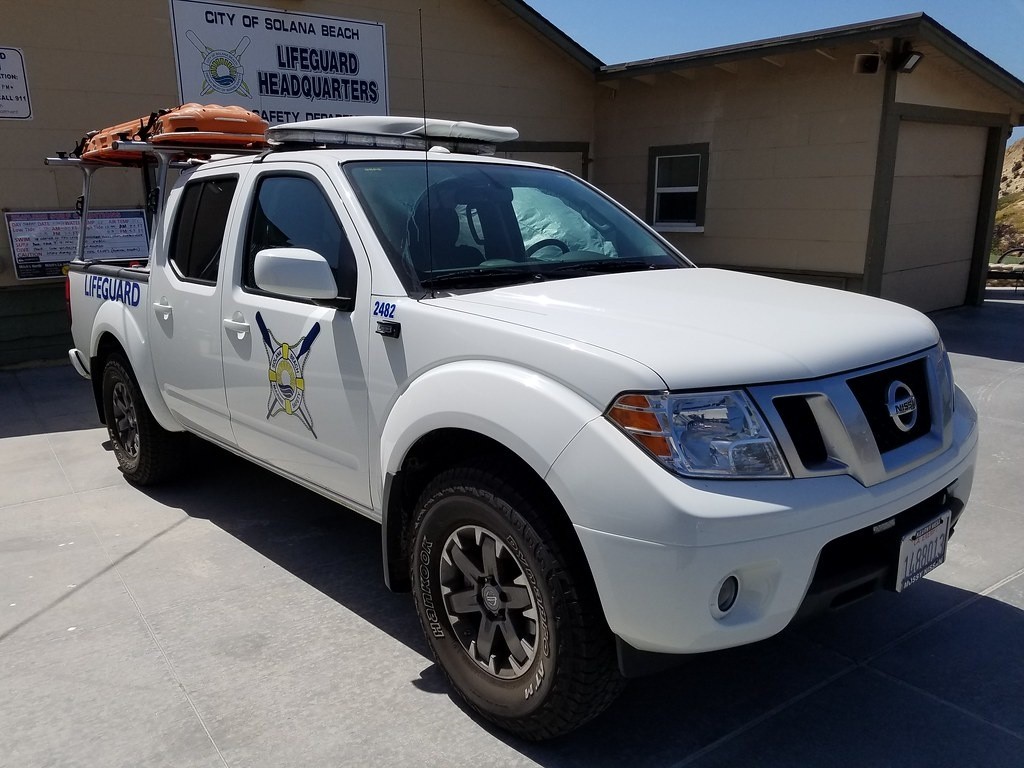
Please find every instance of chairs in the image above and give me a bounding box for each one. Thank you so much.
[406,206,486,282]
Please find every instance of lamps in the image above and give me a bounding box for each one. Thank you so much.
[900,40,926,74]
[852,46,890,74]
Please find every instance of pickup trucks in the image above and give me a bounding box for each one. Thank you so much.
[45,102,981,747]
